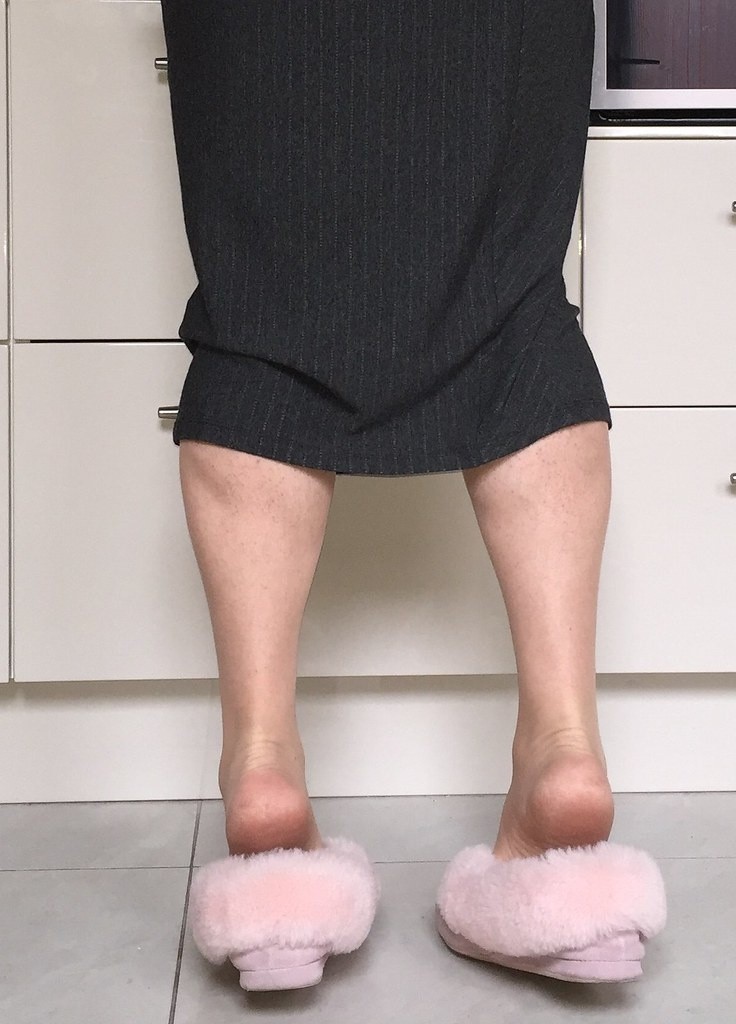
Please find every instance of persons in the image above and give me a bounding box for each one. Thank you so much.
[159,0,668,860]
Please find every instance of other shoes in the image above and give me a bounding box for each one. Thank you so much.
[434,841,666,984]
[193,837,377,990]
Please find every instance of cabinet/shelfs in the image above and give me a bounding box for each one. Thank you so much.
[0,0,736,806]
